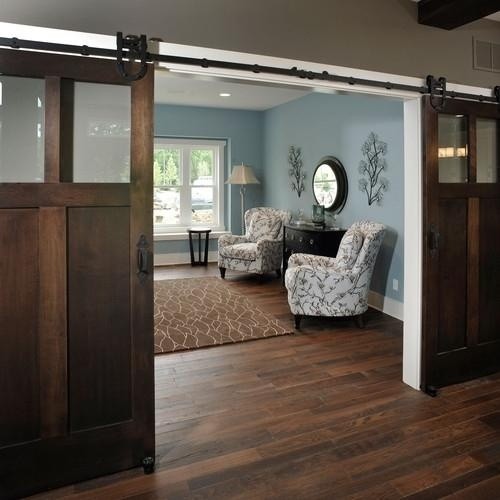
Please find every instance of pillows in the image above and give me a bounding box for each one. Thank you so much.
[246,211,282,243]
[333,224,364,270]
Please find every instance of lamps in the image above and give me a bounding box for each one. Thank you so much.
[224,162,261,235]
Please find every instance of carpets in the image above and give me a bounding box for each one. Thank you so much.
[154,276,295,355]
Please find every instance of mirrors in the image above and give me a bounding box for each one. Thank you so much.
[312,156,348,217]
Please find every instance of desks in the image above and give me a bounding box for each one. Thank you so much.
[187,228,211,267]
[283,222,347,286]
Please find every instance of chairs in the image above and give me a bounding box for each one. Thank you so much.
[285,220,387,332]
[218,207,293,282]
